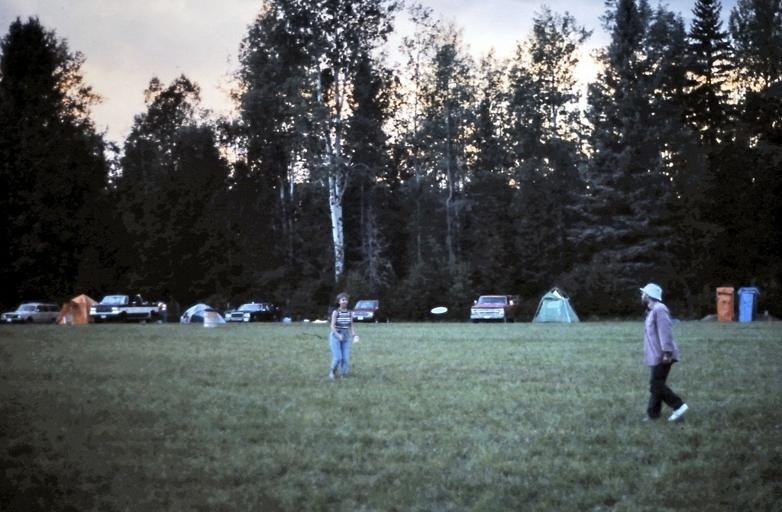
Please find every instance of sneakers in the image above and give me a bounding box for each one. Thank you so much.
[668,403,688,421]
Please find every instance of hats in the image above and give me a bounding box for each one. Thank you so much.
[640,283,662,302]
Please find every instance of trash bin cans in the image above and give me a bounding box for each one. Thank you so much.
[717,287,734,322]
[737,287,760,322]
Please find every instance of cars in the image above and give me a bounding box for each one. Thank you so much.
[352,300,384,321]
[225,302,275,322]
[470,294,517,321]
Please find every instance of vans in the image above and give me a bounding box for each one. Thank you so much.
[3,302,59,323]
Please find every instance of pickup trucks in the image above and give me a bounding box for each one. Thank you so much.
[89,294,161,324]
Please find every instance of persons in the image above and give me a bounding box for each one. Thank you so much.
[638,282,690,424]
[329,291,359,380]
[132,293,149,324]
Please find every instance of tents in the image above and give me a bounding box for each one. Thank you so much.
[531,286,580,323]
[55,293,99,324]
[179,303,227,325]
